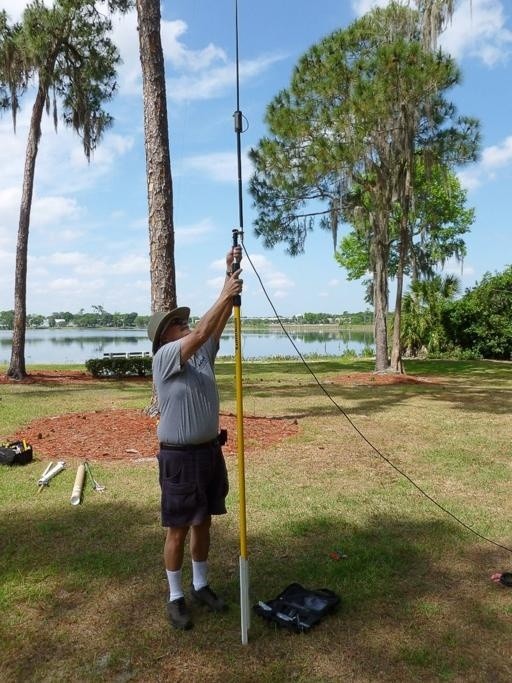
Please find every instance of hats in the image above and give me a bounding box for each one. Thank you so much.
[147,305,190,356]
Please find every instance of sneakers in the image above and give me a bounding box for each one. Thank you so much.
[166,592,194,630]
[190,581,229,612]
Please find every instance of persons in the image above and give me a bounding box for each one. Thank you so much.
[147,243,244,631]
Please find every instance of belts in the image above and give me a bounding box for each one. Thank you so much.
[160,436,223,451]
